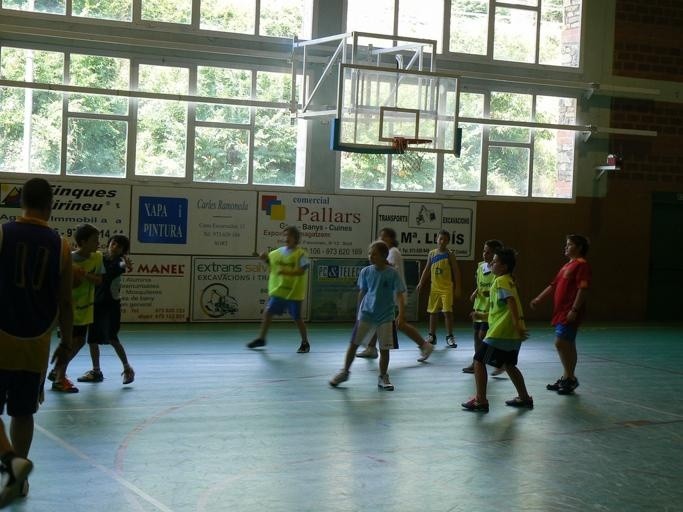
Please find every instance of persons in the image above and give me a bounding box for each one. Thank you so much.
[415,229,462,348]
[462,238,505,376]
[326,238,407,392]
[529,232,592,395]
[78,233,134,385]
[0,176,77,511]
[461,245,534,412]
[245,224,311,354]
[45,221,136,394]
[355,227,436,362]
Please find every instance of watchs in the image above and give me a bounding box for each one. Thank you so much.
[571,306,579,313]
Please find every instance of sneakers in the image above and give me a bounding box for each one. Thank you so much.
[331,369,350,385]
[417,344,432,361]
[461,396,489,411]
[121,368,134,384]
[379,374,394,389]
[0,452,33,507]
[463,362,476,372]
[77,371,104,382]
[505,396,533,408]
[357,346,377,357]
[547,377,579,393]
[48,369,79,393]
[297,343,310,354]
[246,338,265,348]
[425,333,457,348]
[491,368,506,375]
